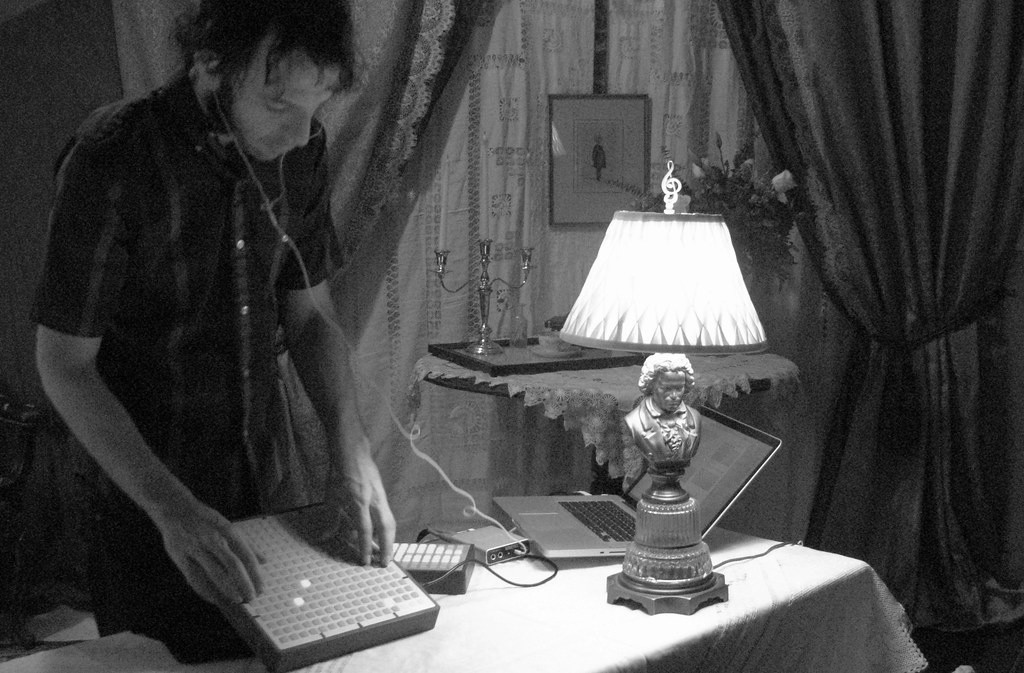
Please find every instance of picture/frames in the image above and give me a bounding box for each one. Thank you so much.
[547,92,651,227]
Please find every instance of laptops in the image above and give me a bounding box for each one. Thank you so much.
[492,406,782,558]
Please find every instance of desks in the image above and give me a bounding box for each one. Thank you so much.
[0,526,872,673]
[419,353,798,495]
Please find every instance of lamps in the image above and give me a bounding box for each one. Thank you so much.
[553,156,770,613]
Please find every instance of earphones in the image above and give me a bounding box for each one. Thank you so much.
[207,60,217,73]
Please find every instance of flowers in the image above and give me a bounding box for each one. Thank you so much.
[648,129,808,294]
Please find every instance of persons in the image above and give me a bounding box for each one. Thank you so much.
[625,351,700,470]
[34,0,395,658]
